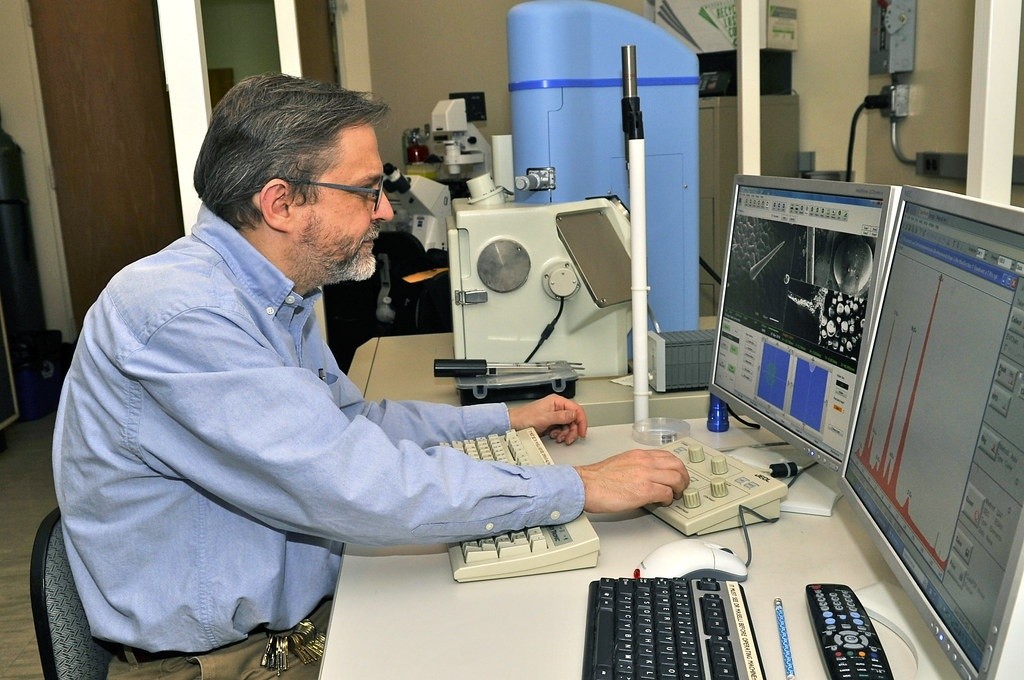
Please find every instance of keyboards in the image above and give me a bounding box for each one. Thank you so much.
[581,578,767,680]
[439,426,602,582]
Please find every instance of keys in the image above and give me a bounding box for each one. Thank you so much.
[260,620,327,677]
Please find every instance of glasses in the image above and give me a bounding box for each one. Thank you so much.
[250,177,384,212]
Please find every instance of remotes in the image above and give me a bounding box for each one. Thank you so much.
[806,584,894,680]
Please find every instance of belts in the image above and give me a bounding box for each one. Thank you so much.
[112,644,190,663]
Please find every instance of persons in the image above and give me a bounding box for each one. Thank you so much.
[53,73,691,680]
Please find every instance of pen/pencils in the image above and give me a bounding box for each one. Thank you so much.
[773,597,795,679]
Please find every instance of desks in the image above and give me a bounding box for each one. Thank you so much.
[320,415,1024,680]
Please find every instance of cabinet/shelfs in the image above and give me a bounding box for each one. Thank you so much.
[699,95,798,315]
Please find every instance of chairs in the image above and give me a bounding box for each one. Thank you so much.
[30,506,112,680]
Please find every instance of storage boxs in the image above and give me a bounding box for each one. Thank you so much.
[694,48,794,101]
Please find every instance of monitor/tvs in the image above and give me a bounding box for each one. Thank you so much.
[835,184,1024,680]
[710,174,904,517]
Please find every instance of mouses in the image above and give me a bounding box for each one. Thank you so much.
[633,538,748,582]
